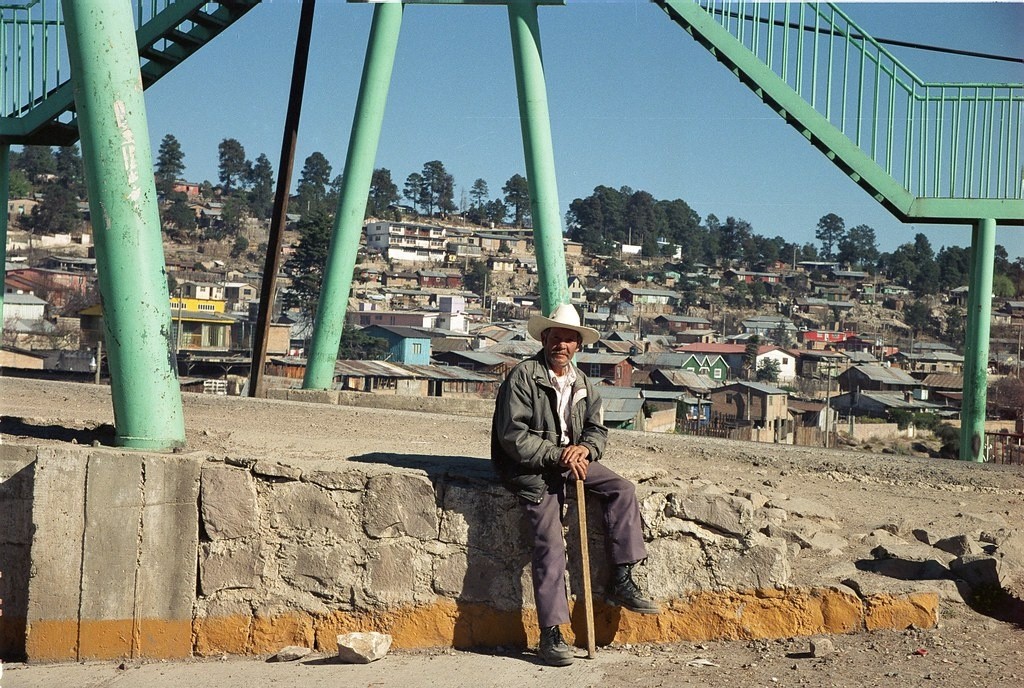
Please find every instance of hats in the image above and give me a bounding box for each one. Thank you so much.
[527,303,600,345]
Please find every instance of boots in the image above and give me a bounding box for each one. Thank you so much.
[539,626,574,666]
[606,565,660,613]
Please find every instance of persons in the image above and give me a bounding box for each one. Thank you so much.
[491,304,661,665]
